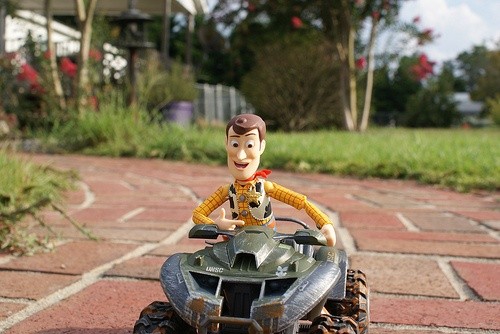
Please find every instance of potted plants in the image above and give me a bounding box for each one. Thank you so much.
[148,54,198,127]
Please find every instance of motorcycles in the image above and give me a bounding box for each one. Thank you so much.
[133,216,371,334]
